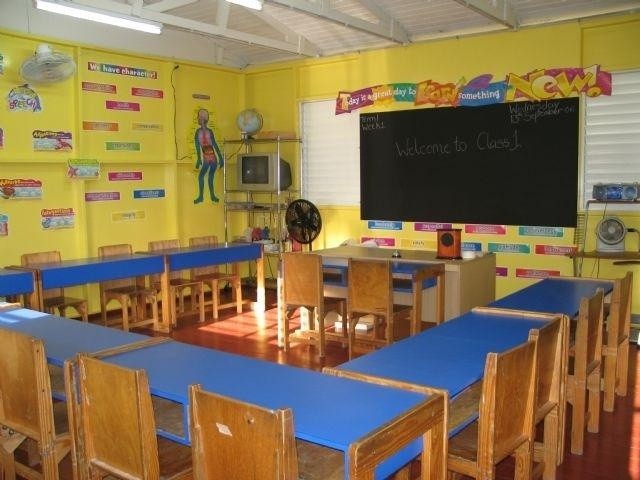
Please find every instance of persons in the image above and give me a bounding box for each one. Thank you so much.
[190,106,226,207]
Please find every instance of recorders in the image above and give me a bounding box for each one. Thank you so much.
[592,182,640,203]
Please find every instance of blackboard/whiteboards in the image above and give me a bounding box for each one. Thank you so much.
[359,96,579,228]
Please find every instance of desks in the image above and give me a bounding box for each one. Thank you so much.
[135,240,269,318]
[10,250,177,333]
[318,307,562,397]
[62,334,445,479]
[274,253,447,354]
[1,300,159,373]
[471,274,618,314]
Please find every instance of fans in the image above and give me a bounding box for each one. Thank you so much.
[21,42,74,86]
[285,198,322,252]
[594,216,626,253]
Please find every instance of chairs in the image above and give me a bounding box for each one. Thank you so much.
[189,236,245,321]
[0,328,67,478]
[447,315,568,480]
[345,257,414,358]
[96,242,161,336]
[181,380,300,480]
[20,250,89,322]
[568,287,608,459]
[602,269,632,415]
[279,252,346,356]
[70,353,189,479]
[145,238,209,327]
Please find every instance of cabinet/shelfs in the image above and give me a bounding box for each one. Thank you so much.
[223,135,303,281]
[571,200,640,324]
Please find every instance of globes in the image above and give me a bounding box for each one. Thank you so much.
[236,107,264,140]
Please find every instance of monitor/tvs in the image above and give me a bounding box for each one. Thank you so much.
[237,152,292,191]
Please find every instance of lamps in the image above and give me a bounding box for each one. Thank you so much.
[32,0,165,35]
